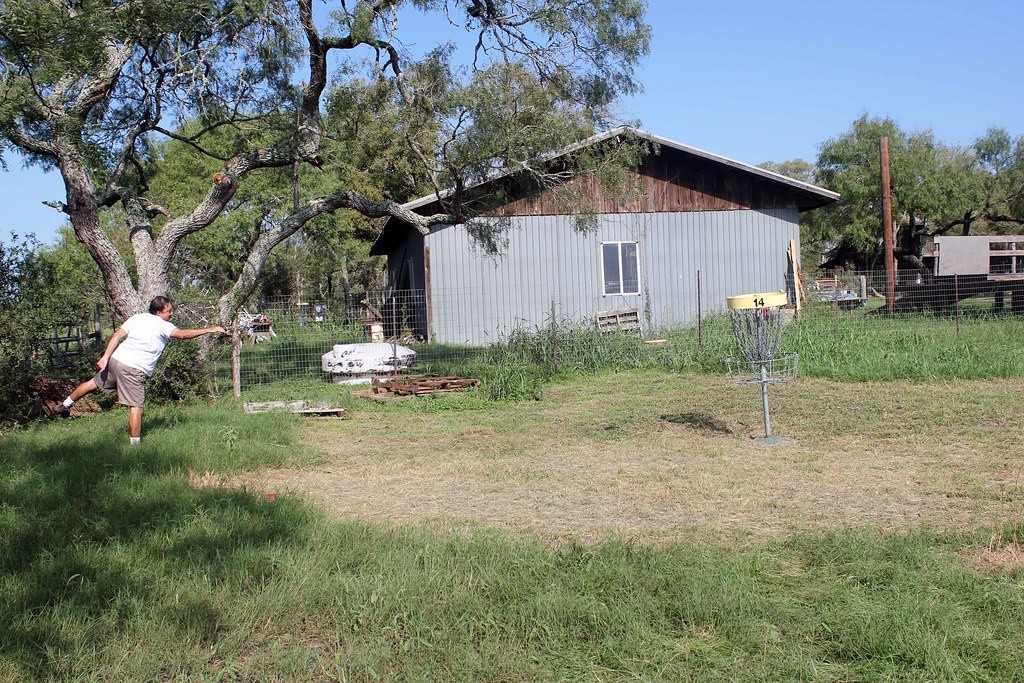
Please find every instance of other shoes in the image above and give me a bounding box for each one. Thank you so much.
[53,405,70,418]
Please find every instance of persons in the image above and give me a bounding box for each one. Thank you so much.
[53,295,227,445]
